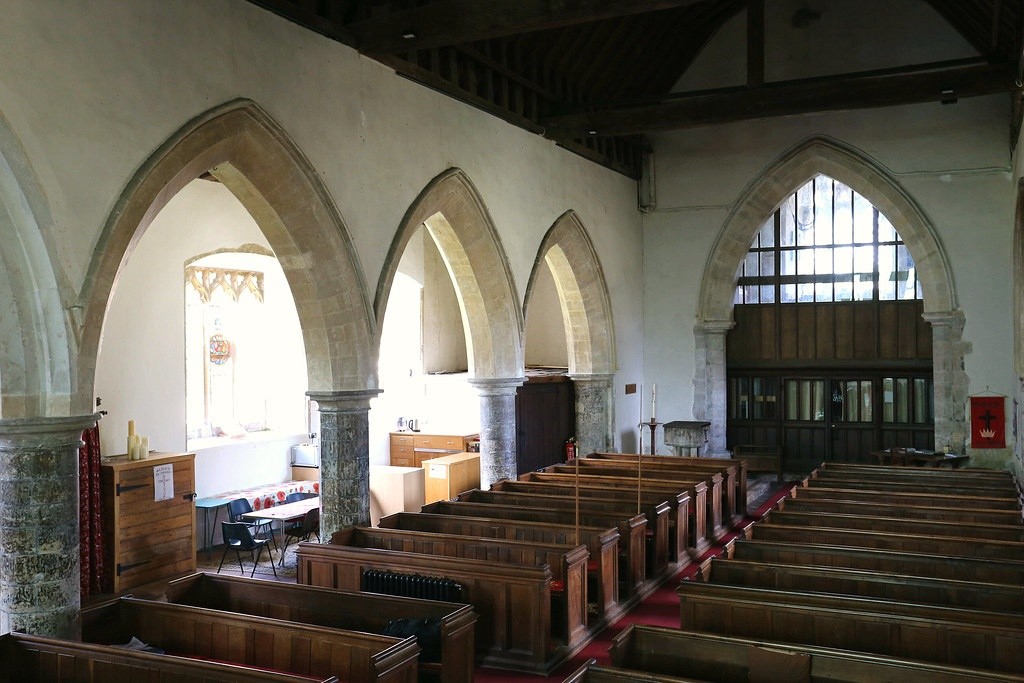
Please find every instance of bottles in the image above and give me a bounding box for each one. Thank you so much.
[127,435,134,460]
[398,417,407,432]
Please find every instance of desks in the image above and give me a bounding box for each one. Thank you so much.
[241,497,322,567]
[872,447,968,470]
[195,499,231,560]
[208,481,320,548]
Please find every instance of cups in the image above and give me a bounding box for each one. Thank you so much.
[409,418,421,432]
[132,444,140,460]
[140,445,148,459]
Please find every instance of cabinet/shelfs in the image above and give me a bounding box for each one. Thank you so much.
[100,453,197,595]
[292,466,320,480]
[369,430,480,528]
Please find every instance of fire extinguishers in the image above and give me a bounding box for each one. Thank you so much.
[565,436,575,463]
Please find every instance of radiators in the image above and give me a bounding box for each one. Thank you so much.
[361,571,465,604]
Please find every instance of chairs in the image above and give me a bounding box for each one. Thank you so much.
[217,492,320,578]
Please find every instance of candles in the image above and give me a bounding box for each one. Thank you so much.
[652,383,655,417]
[126,421,150,461]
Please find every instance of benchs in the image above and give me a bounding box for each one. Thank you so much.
[0,452,1024,683]
